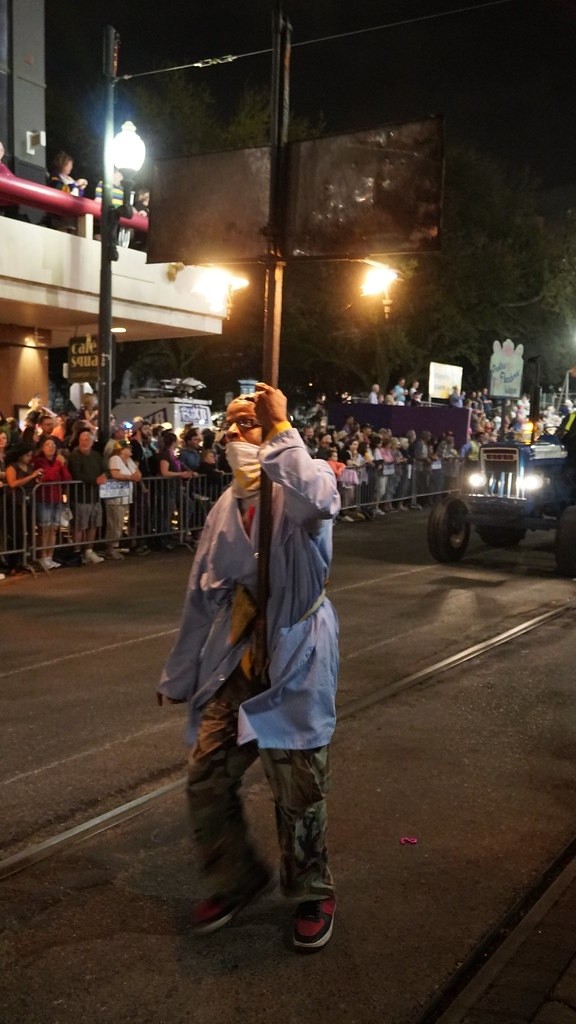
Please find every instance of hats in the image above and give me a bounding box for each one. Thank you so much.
[360,423,374,428]
[113,439,134,449]
[132,416,144,424]
[446,430,456,437]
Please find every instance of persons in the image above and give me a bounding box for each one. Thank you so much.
[449,384,576,464]
[95,170,150,248]
[301,415,459,527]
[48,150,88,233]
[0,141,16,218]
[154,382,342,946]
[368,378,424,407]
[0,394,234,581]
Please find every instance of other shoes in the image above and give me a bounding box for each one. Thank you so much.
[332,496,441,526]
[152,541,176,553]
[0,573,5,580]
[86,551,104,564]
[8,566,23,576]
[19,565,31,574]
[40,557,55,570]
[128,544,151,556]
[106,550,126,560]
[48,557,61,569]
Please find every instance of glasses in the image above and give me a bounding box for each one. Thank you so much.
[44,444,56,450]
[219,418,265,430]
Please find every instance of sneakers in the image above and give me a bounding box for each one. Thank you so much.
[292,893,337,948]
[189,868,277,932]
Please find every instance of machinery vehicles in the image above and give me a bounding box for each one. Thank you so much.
[427,409,576,570]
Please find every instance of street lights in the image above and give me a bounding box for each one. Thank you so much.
[98,26,147,455]
